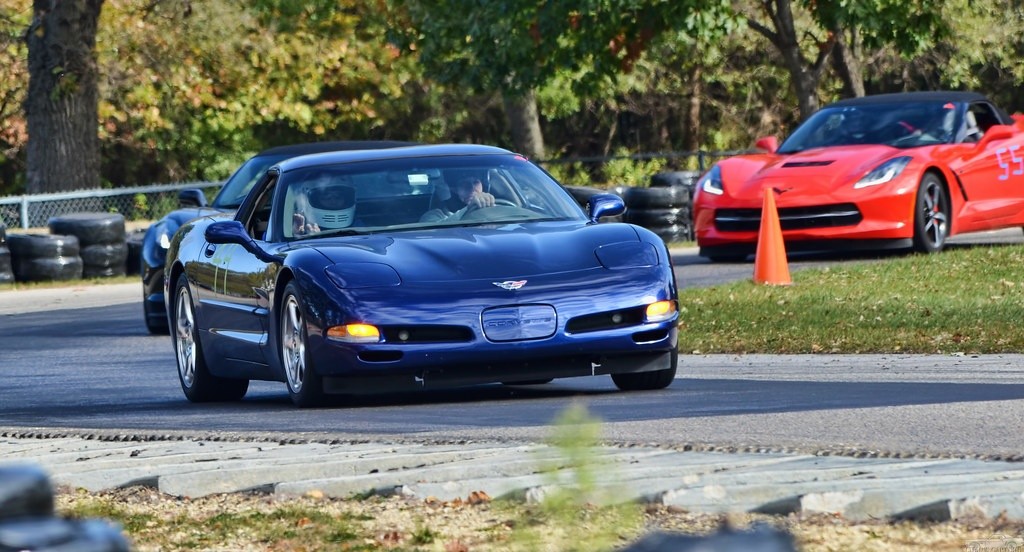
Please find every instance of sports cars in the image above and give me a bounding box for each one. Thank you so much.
[163,140,679,405]
[141,141,418,337]
[692,88,1024,252]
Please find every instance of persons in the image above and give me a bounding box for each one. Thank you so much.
[262,177,366,240]
[419,168,496,225]
[913,111,985,143]
